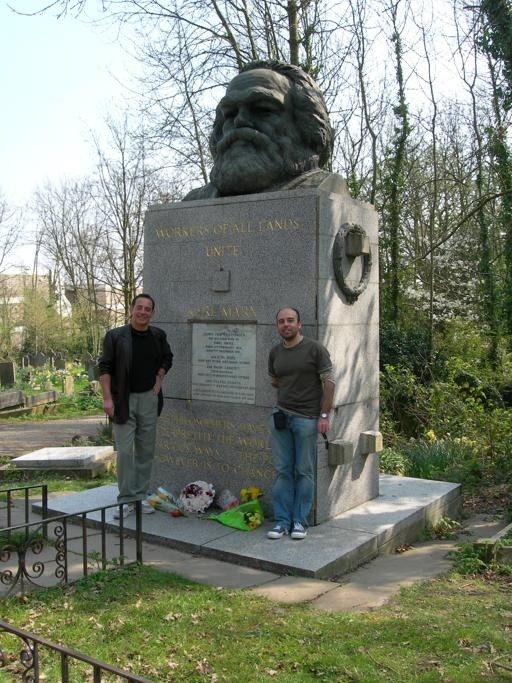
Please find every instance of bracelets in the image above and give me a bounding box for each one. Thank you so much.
[157,372,164,380]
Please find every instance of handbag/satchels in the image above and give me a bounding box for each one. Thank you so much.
[273,410,286,430]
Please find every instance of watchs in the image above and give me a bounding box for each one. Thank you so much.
[320,412,328,419]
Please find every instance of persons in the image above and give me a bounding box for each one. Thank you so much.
[266,306,337,540]
[179,57,354,200]
[97,293,174,520]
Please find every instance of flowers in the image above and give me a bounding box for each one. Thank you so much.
[148,480,263,530]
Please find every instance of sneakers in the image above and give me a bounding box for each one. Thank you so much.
[266,520,308,539]
[113,498,155,519]
